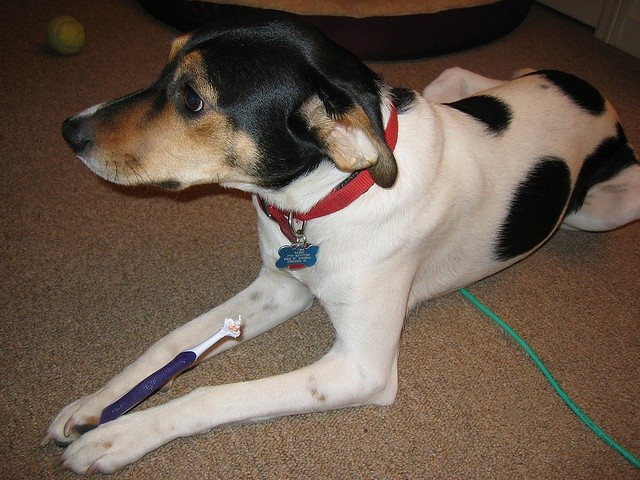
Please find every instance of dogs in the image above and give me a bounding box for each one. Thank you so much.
[38,16,640,477]
